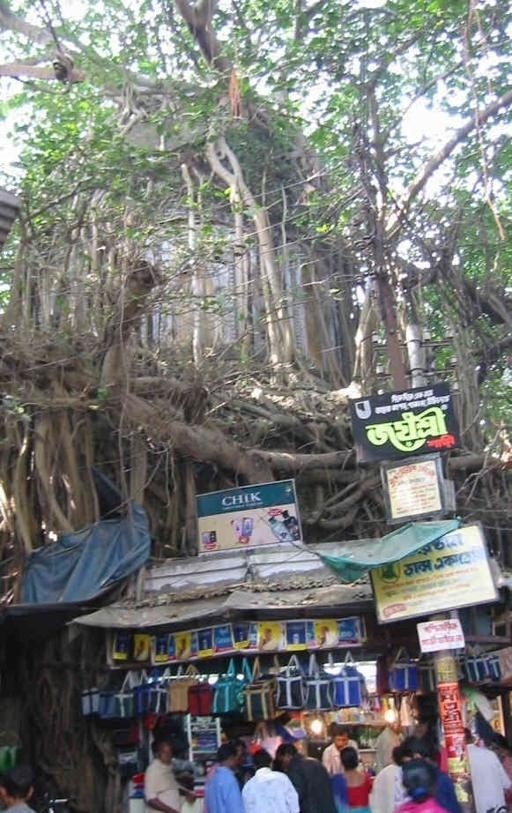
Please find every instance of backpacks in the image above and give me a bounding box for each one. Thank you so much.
[211,654,251,718]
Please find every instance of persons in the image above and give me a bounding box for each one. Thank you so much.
[178,638,189,657]
[316,626,331,644]
[136,639,149,660]
[204,711,512,813]
[144,736,196,813]
[257,628,274,650]
[0,768,41,813]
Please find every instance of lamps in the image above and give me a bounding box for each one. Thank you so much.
[310,711,323,734]
[384,699,395,722]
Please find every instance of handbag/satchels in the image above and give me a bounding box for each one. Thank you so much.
[82,663,212,718]
[274,649,364,711]
[388,646,417,695]
[422,642,500,694]
[240,654,288,723]
